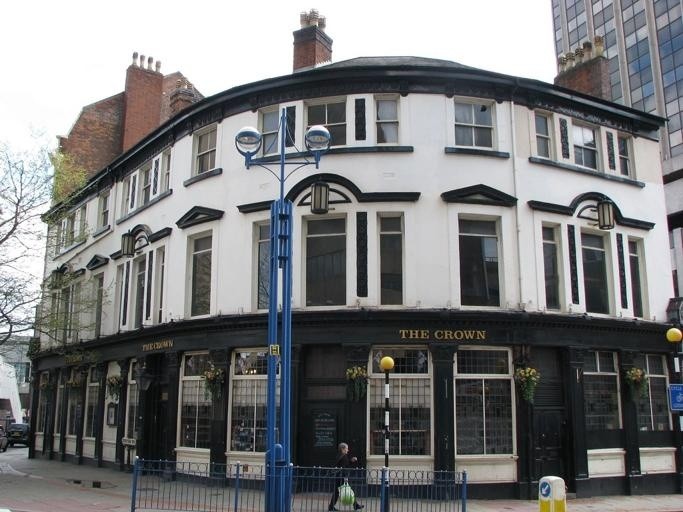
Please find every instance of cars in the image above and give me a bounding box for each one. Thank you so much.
[0,424,8,452]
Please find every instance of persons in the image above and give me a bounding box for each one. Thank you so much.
[328,443,364,512]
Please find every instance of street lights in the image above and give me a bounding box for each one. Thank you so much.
[666,327,683,441]
[229,103,330,512]
[378,356,396,512]
[5,410,11,427]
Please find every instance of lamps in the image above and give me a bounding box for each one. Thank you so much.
[121,233,136,257]
[310,183,329,214]
[52,271,63,289]
[597,199,615,230]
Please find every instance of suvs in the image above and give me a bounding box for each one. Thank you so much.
[5,421,30,449]
